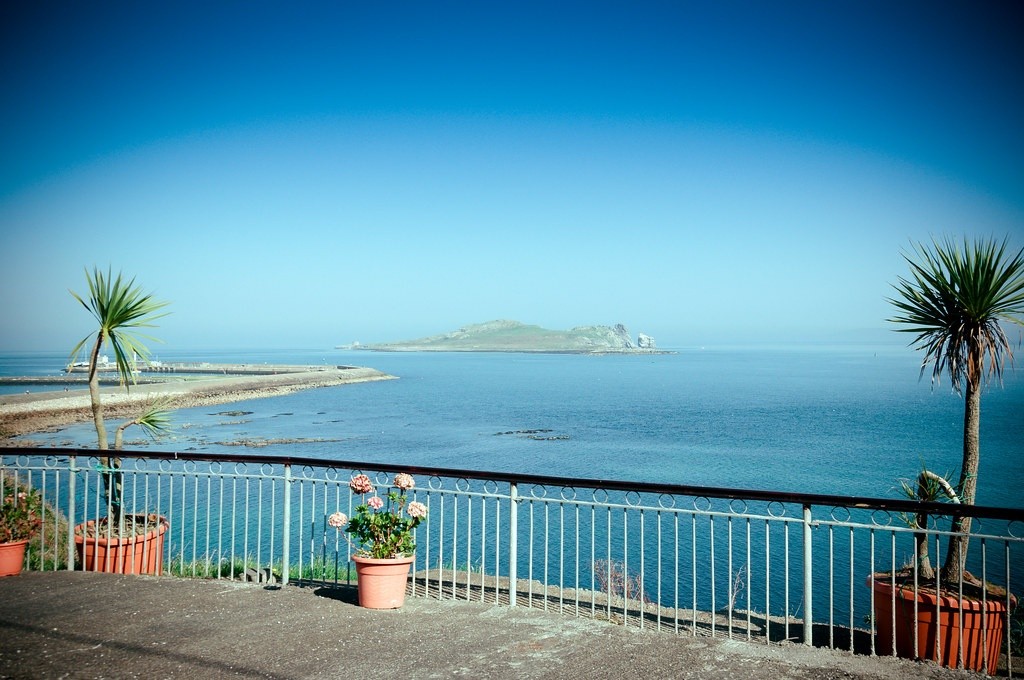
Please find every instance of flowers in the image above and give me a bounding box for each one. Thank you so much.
[329,473,428,558]
[0,475,45,544]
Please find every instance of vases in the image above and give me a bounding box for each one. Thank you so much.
[351,555,415,609]
[0,540,27,577]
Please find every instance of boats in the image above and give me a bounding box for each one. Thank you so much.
[66,342,110,369]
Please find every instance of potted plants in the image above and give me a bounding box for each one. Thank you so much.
[66,263,180,575]
[866,232,1024,675]
